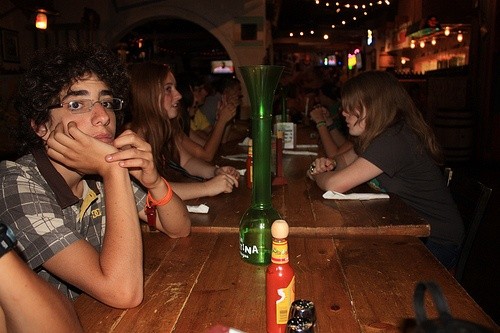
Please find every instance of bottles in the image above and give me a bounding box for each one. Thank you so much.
[237,64,287,265]
[286,300,319,333]
[266,220,296,333]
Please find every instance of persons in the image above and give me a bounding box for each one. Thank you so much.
[304,84,341,128]
[115,65,240,200]
[306,71,473,269]
[0,221,83,333]
[0,42,191,308]
[199,74,248,140]
[310,107,386,192]
[177,72,239,161]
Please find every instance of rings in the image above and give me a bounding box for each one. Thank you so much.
[312,166,315,168]
[311,168,314,172]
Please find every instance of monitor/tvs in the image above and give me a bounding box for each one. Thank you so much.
[211,60,234,75]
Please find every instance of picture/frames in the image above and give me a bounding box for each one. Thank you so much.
[0,27,21,64]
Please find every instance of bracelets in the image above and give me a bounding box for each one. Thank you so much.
[327,122,335,132]
[317,122,326,127]
[146,177,172,226]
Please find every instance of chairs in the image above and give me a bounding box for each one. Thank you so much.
[445,167,493,279]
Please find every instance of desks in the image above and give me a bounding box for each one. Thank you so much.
[70,233,500,333]
[137,123,431,238]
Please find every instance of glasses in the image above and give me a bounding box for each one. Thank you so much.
[45,98,124,114]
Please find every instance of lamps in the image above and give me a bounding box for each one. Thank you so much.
[34,8,48,30]
